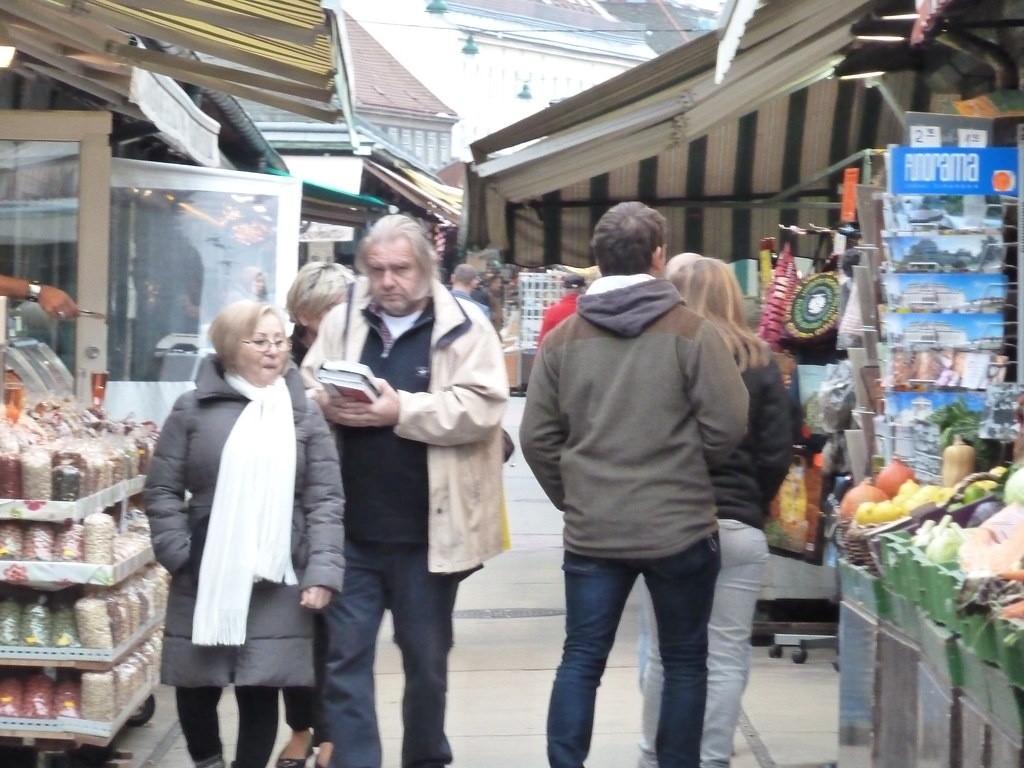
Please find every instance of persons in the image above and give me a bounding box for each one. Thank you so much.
[239,265,268,305]
[262,259,516,766]
[522,199,752,768]
[628,254,806,765]
[536,274,589,348]
[0,273,80,321]
[304,216,512,768]
[433,260,508,339]
[143,299,347,768]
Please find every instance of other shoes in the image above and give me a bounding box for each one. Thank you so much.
[315,753,330,768]
[276,735,314,768]
[194,753,226,768]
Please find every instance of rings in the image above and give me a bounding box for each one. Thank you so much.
[57,311,64,317]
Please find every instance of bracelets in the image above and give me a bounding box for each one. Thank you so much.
[26,280,41,303]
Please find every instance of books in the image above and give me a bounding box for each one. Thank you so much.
[312,357,382,404]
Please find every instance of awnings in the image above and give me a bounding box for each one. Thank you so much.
[468,0,973,270]
[261,165,399,227]
[351,146,469,234]
[0,1,359,169]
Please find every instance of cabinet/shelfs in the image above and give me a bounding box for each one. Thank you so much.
[1,473,165,747]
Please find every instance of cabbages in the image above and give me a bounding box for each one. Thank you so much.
[928,469,1024,562]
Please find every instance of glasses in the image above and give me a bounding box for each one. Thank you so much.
[241,337,293,352]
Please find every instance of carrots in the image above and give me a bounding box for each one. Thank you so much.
[995,570,1023,623]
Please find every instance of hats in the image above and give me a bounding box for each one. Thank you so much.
[562,275,586,288]
[479,270,503,285]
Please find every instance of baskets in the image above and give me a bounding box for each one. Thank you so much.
[836,518,903,570]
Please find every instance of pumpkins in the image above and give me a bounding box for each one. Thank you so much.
[840,458,915,522]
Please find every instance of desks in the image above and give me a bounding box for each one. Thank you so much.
[835,555,1024,768]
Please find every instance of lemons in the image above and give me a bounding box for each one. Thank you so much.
[855,467,1005,526]
